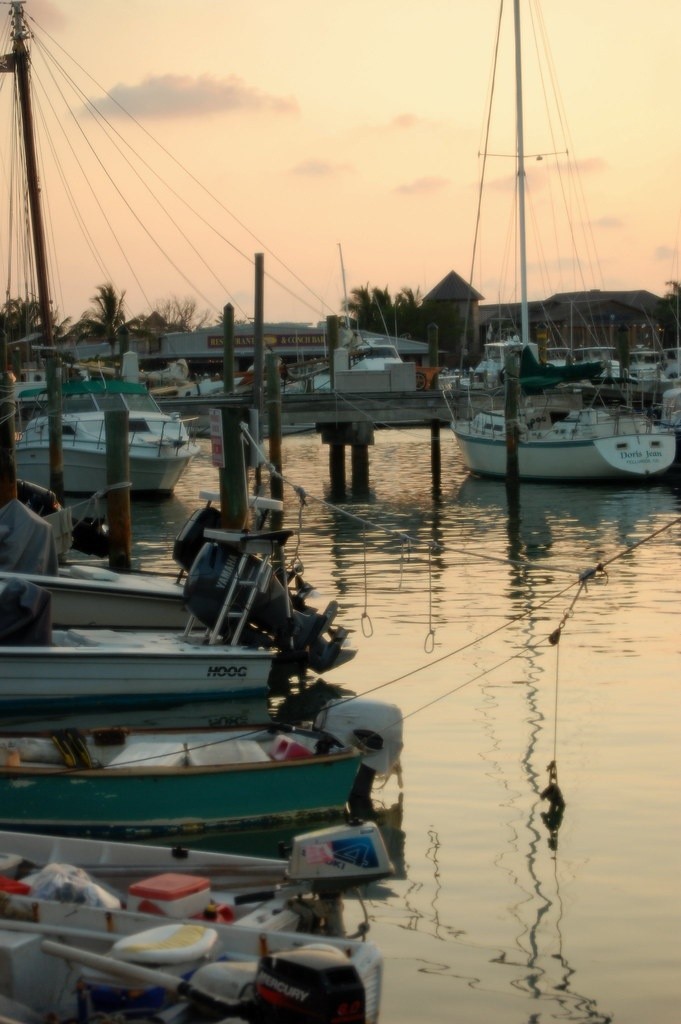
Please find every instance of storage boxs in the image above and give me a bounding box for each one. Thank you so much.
[124,873,211,922]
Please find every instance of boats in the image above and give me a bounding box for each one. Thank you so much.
[0,494,247,624]
[442,389,677,480]
[0,499,353,706]
[16,380,194,491]
[99,344,404,393]
[449,11,606,383]
[546,347,680,383]
[0,817,396,1024]
[0,695,403,823]
